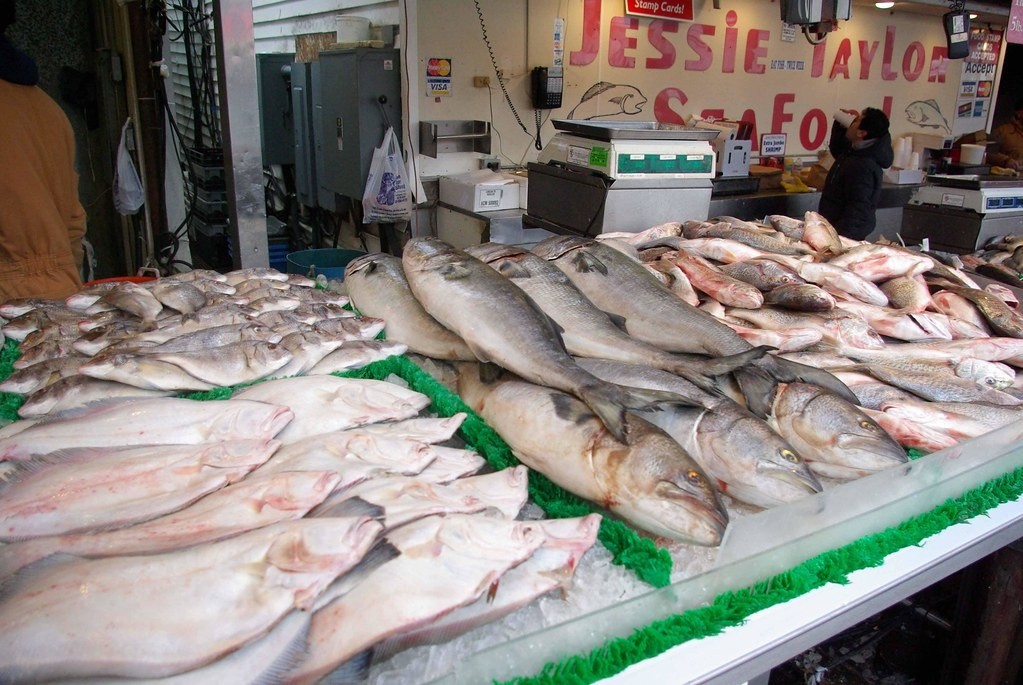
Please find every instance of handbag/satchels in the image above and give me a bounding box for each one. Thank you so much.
[109,145,145,218]
[362,126,412,224]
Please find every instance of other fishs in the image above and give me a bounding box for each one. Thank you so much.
[0,213,1023,685]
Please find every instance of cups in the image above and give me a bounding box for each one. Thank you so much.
[893,136,919,171]
[833,110,857,129]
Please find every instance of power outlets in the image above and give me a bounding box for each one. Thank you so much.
[474,76,489,87]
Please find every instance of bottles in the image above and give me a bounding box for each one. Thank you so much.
[792,158,802,177]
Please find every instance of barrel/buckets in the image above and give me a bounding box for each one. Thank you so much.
[335,15,370,43]
[285,248,370,280]
[960,144,986,165]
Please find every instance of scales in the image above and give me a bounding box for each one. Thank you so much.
[908,173,1023,216]
[536,118,723,178]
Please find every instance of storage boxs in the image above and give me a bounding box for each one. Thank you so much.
[439,168,528,213]
[709,139,752,180]
[182,147,229,268]
[225,219,290,272]
[882,169,923,184]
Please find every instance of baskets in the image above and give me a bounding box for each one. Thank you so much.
[183,145,229,270]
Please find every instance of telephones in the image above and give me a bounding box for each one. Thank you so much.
[532,66,564,110]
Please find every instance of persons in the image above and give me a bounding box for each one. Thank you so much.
[986,104,1023,172]
[0,37,88,307]
[818,107,894,242]
